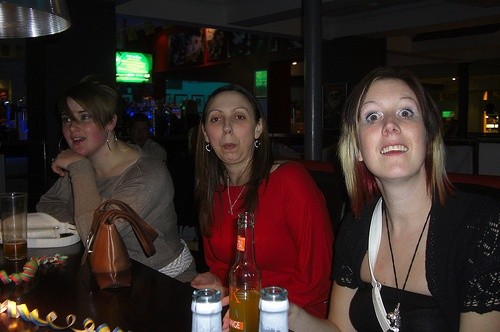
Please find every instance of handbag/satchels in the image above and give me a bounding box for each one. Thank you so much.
[81,199,159,290]
[0,212,80,249]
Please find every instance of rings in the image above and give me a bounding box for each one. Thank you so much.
[51,158,56,162]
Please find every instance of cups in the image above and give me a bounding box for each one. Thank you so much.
[0,192,28,261]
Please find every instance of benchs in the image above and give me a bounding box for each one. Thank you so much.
[286,158,500,243]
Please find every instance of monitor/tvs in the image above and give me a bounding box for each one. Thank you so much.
[115,50,154,84]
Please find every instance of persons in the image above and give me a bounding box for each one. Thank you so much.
[36,80,197,285]
[119,99,200,229]
[188,84,334,320]
[0,91,14,122]
[220,66,500,332]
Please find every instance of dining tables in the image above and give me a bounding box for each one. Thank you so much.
[0,233,295,332]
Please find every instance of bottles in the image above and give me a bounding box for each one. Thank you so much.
[229,212,262,332]
[191,288,222,332]
[258,287,289,332]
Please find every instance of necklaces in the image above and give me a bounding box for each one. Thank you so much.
[226,171,246,214]
[381,199,432,329]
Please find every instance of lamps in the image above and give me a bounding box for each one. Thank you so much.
[111,17,155,85]
[0,0,72,39]
